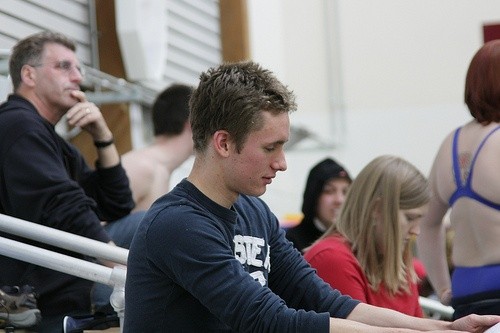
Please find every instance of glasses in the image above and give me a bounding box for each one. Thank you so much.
[33,61,80,71]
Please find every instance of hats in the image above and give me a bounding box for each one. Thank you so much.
[302,158,352,220]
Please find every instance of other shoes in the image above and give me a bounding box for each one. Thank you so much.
[0,283,41,327]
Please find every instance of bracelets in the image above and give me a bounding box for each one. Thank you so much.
[92,137,113,148]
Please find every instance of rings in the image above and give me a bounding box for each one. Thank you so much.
[84,107,89,113]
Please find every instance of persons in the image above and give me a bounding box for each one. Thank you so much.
[0,40,500,333]
